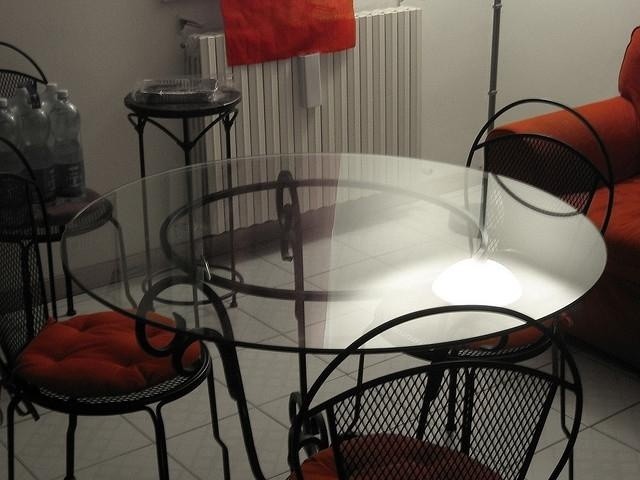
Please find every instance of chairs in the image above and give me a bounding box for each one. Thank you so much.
[346,95,615,480]
[484,23,640,370]
[0,42,142,329]
[1,140,230,480]
[287,306,584,478]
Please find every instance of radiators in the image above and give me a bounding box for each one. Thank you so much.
[183,3,422,234]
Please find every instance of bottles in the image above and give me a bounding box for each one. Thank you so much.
[0,80,86,209]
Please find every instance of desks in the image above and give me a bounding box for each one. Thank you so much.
[58,151,606,479]
[124,86,242,310]
